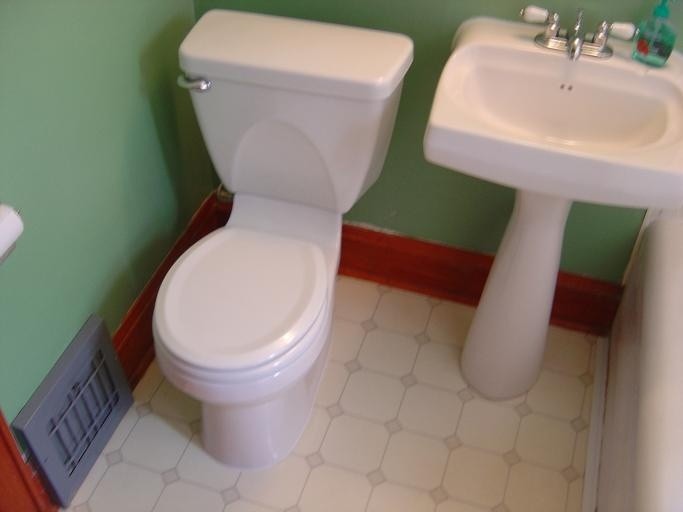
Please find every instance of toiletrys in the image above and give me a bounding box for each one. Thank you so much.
[630,0,677,69]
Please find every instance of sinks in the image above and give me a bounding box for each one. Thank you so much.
[422,16,683,212]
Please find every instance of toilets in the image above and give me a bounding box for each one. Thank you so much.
[151,8,416,474]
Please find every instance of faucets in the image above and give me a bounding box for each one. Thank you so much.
[568,9,586,62]
